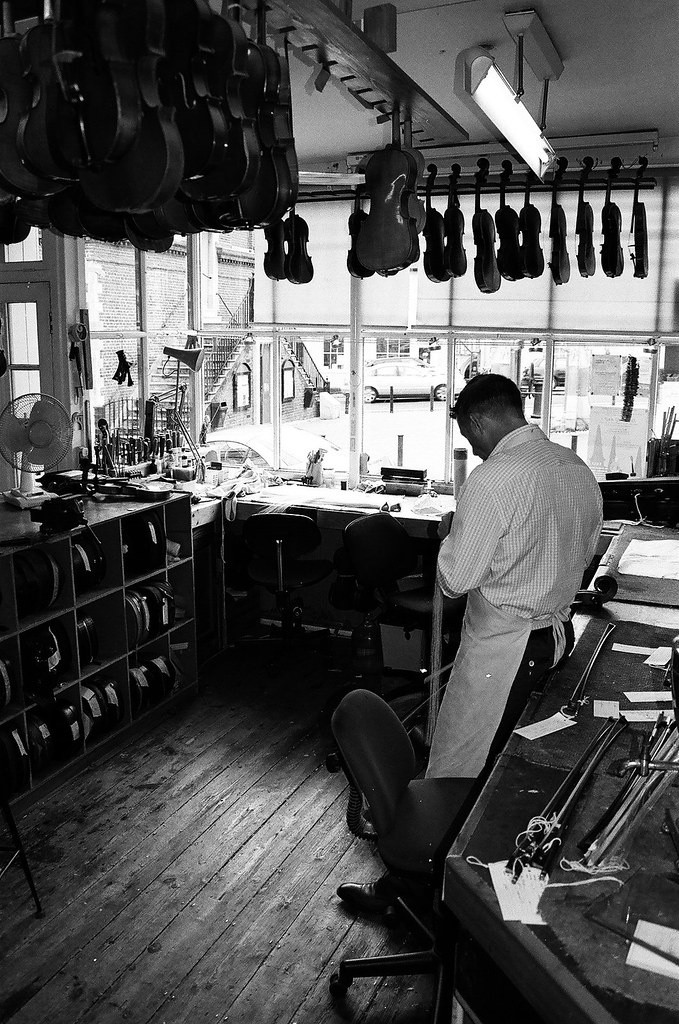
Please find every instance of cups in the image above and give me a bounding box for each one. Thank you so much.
[323,468,336,487]
[306,463,323,485]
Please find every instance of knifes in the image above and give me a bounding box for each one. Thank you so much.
[111,430,194,466]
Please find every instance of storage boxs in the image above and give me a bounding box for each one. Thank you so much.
[376,617,429,677]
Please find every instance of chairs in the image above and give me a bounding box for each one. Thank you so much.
[239,513,338,674]
[325,688,480,1024]
[343,511,467,703]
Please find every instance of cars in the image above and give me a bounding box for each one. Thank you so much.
[530,354,568,389]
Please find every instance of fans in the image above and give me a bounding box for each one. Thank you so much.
[0,393,73,509]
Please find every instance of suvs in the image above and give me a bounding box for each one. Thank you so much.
[363,356,466,403]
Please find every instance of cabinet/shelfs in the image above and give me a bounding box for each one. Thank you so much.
[1,479,201,830]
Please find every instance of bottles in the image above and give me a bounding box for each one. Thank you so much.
[341,480,347,490]
[167,447,189,469]
[453,447,468,500]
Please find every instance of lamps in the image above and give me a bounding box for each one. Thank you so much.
[162,347,204,412]
[462,47,560,183]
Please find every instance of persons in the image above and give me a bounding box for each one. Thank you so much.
[337,373,604,913]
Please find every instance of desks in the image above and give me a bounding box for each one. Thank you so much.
[191,478,679,1024]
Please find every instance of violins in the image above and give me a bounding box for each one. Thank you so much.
[575,155,597,277]
[518,171,545,279]
[472,157,502,295]
[0,0,301,254]
[495,160,524,282]
[401,117,427,235]
[356,110,420,271]
[346,160,467,284]
[627,156,650,280]
[283,205,314,284]
[263,219,287,281]
[549,156,571,286]
[600,157,624,277]
